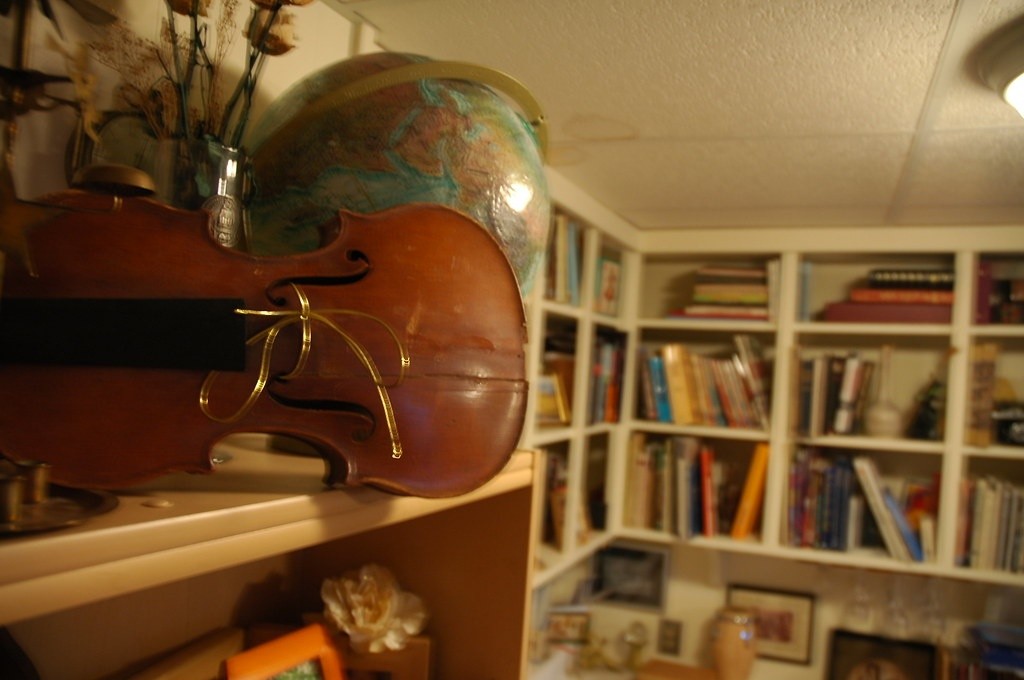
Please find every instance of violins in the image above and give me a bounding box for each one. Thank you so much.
[0,191,533,499]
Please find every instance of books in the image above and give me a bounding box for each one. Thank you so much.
[787,353,946,564]
[532,210,626,571]
[957,249,1023,574]
[626,260,772,538]
[822,300,950,324]
[866,265,953,289]
[849,287,952,306]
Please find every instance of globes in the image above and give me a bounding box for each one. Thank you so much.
[241,52,554,304]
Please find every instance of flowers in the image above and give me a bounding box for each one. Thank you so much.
[80,0,312,140]
[321,562,430,653]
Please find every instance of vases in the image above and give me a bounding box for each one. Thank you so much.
[172,141,241,248]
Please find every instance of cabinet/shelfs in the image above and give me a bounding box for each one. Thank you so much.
[529,174,638,588]
[0,447,548,680]
[617,223,1024,586]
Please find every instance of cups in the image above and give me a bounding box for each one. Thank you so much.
[0,475,27,523]
[15,460,51,505]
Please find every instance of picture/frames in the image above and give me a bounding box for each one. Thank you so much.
[657,618,681,656]
[547,612,589,644]
[591,547,669,614]
[220,625,342,680]
[726,581,816,666]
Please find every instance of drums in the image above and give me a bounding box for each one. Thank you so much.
[710,610,756,680]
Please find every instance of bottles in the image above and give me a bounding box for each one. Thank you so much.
[202,146,245,252]
